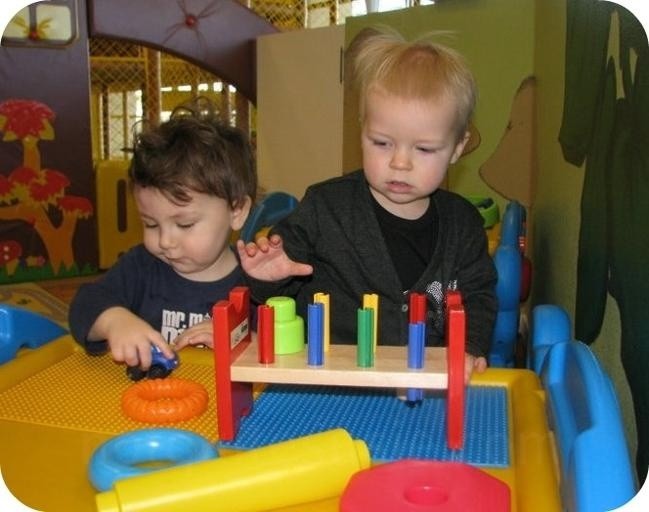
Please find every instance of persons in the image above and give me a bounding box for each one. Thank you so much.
[65,111,252,371]
[234,33,500,384]
